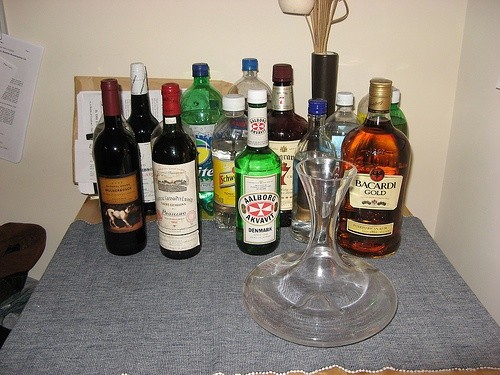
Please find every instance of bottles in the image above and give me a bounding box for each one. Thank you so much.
[180,63,224,216]
[228,57,272,116]
[324,92,360,160]
[290,98,337,243]
[266,63,308,228]
[235,89,281,256]
[211,94,249,232]
[336,78,412,259]
[127,63,162,216]
[93,79,147,256]
[151,83,202,260]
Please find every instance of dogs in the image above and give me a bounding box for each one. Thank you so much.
[105,204,135,228]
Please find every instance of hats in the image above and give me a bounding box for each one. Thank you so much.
[0,223,47,304]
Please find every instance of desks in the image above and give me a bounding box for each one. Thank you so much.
[0,199,500,375]
